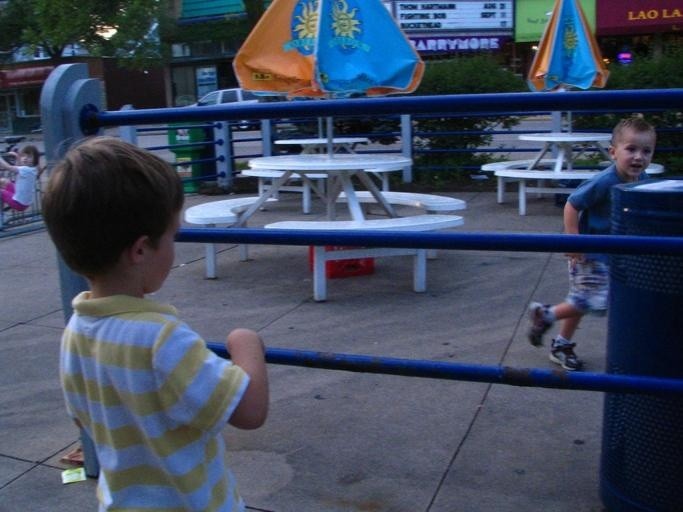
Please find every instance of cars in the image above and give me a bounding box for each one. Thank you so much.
[173,87,288,137]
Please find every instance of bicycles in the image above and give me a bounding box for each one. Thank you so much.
[0,126,45,180]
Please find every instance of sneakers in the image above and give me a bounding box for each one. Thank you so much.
[523,301,552,349]
[547,338,582,373]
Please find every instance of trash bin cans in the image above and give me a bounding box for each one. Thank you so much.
[598,177,683,512]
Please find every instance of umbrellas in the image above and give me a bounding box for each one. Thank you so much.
[528,1,610,136]
[232,1,427,210]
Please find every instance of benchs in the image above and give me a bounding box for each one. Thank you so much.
[479,159,566,205]
[240,169,403,211]
[334,189,466,259]
[494,169,604,216]
[599,160,665,178]
[263,214,464,301]
[183,196,279,280]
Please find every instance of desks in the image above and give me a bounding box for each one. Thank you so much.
[274,138,369,154]
[235,154,415,230]
[518,133,614,172]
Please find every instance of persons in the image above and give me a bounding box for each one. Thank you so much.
[0,145,38,211]
[526,112,657,371]
[40,134,269,512]
